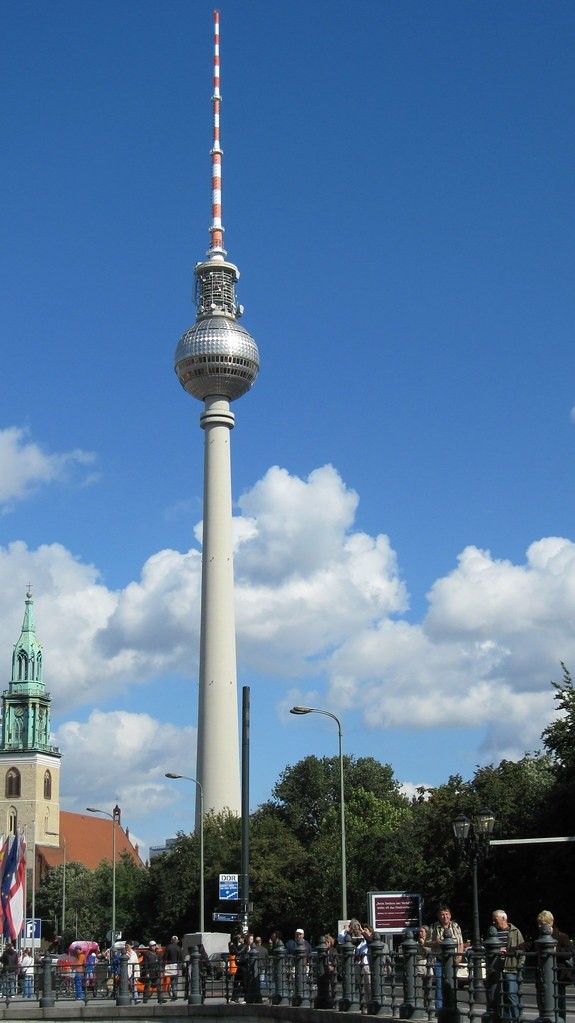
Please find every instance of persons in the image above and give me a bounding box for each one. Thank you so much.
[424,906,464,1017]
[226,932,269,1003]
[286,928,311,997]
[500,910,573,1023]
[324,934,338,1009]
[182,946,197,1001]
[337,919,361,991]
[86,952,97,992]
[487,909,527,1023]
[270,931,292,985]
[72,946,86,1000]
[19,950,34,998]
[123,940,141,1005]
[418,925,434,1010]
[142,940,168,1004]
[162,936,183,1002]
[0,943,18,998]
[196,943,213,998]
[345,922,374,1014]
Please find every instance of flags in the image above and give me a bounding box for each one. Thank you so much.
[0,831,27,941]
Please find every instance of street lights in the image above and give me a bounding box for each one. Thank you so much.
[451,805,496,1004]
[289,705,348,921]
[85,806,117,931]
[45,831,66,931]
[166,772,212,934]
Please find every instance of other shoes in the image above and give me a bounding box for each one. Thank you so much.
[158,999,167,1003]
[3,994,15,998]
[230,998,247,1004]
[143,999,148,1003]
[135,999,141,1005]
[21,995,31,998]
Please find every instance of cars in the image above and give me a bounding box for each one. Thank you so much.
[0,935,178,1001]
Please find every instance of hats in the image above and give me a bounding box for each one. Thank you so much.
[72,946,82,951]
[295,929,304,933]
[149,940,156,945]
